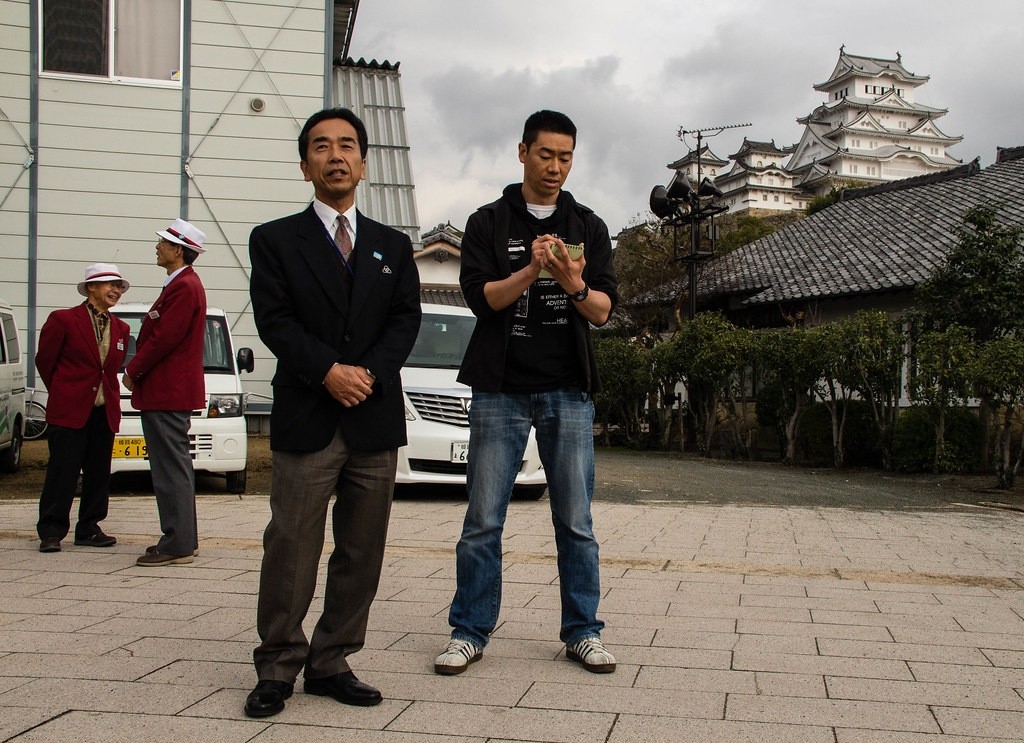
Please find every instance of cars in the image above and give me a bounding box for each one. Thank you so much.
[0,300,26,473]
[80,304,254,495]
[394,303,547,501]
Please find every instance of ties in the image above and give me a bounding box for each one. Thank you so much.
[333,215,352,267]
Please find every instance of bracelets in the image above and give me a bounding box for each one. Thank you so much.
[366,368,375,379]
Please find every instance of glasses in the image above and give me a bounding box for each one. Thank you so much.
[93,281,125,292]
[158,237,173,244]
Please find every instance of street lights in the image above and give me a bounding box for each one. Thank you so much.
[650,132,729,453]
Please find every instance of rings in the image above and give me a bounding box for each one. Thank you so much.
[550,264,553,268]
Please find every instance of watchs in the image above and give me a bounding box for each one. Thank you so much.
[571,286,589,303]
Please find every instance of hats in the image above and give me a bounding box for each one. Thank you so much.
[77,263,130,297]
[156,218,207,254]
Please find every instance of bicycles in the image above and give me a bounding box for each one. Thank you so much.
[23,390,49,441]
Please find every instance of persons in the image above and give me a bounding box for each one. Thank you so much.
[123,218,209,566]
[434,109,617,675]
[242,107,424,719]
[35,262,129,552]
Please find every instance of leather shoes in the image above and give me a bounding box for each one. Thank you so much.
[244,680,293,716]
[303,671,382,705]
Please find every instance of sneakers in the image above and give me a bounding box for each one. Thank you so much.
[434,638,483,674]
[145,540,199,557]
[75,529,117,546]
[136,552,194,567]
[40,537,61,552]
[565,637,616,673]
